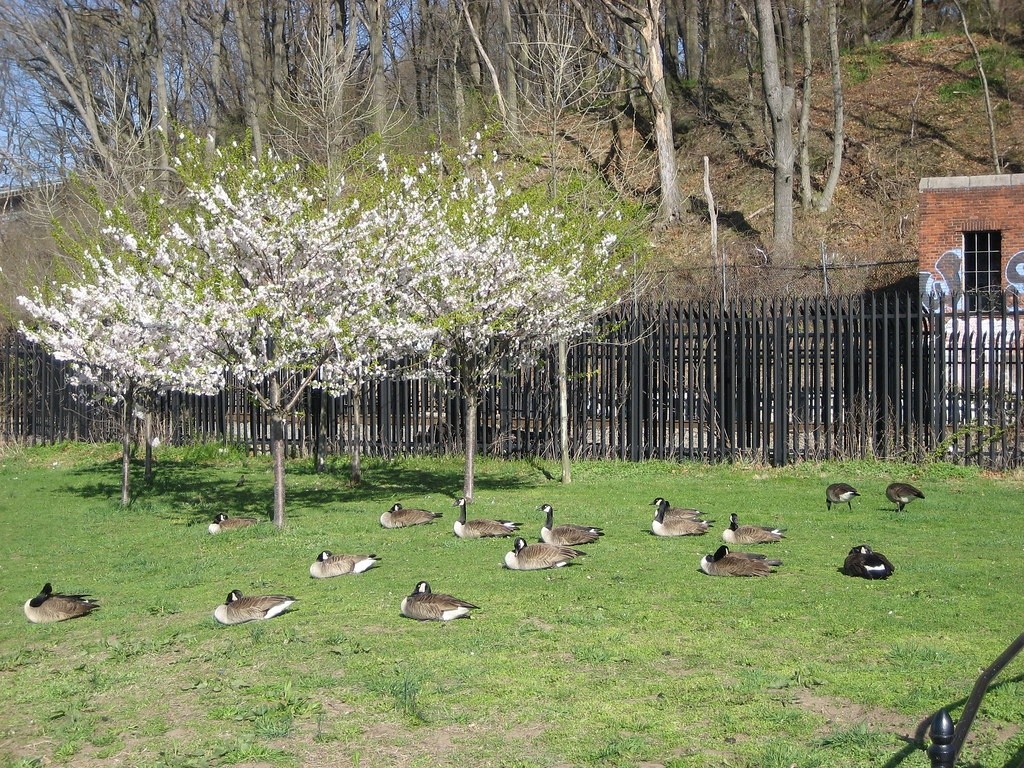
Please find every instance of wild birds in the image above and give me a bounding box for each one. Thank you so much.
[886,483,925,512]
[699,545,782,578]
[535,504,604,547]
[449,497,524,539]
[400,581,480,622]
[825,483,861,512]
[236,475,245,487]
[722,513,788,545]
[208,513,258,535]
[380,503,443,528]
[310,549,382,579]
[649,498,716,537]
[843,545,895,580]
[504,537,587,570]
[214,589,299,626]
[24,583,100,623]
